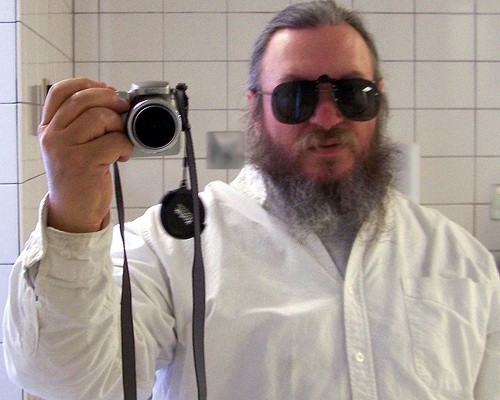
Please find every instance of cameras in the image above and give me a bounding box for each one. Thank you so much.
[115,80,181,159]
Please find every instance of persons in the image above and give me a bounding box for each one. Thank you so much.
[3,0,499,400]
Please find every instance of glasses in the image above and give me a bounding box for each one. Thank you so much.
[252,74,382,124]
